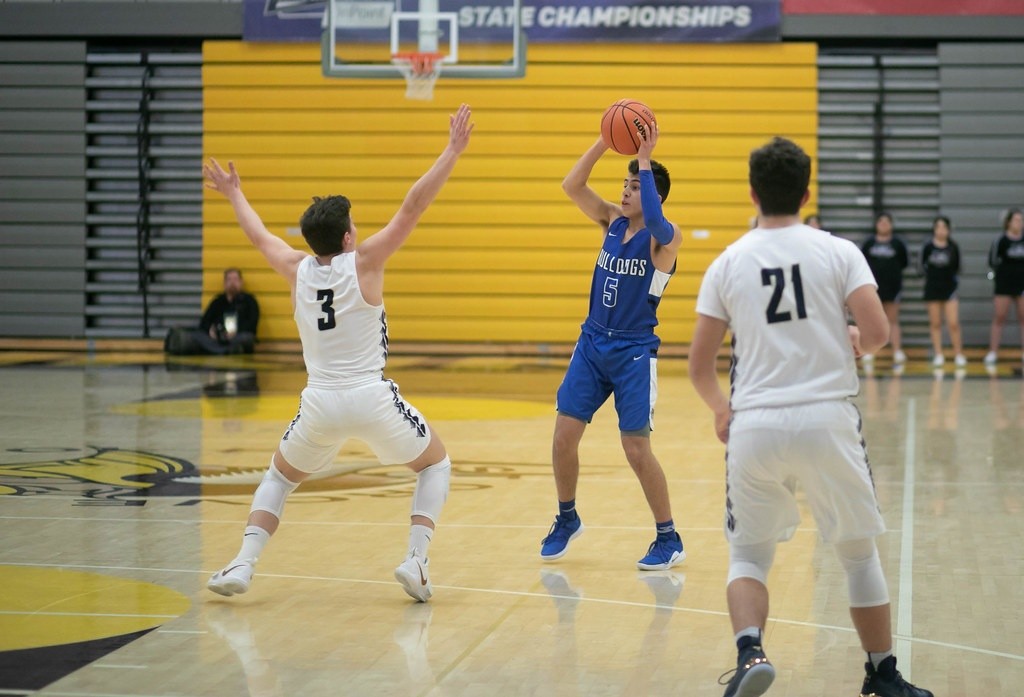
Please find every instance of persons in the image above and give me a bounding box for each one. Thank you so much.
[800,206,910,369]
[983,208,1024,362]
[190,266,260,354]
[919,217,968,366]
[688,135,938,697]
[538,121,692,571]
[201,103,478,603]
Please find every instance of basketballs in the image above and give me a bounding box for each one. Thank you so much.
[600,97,658,155]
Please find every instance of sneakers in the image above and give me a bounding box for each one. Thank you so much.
[858,656,935,697]
[206,557,258,597]
[717,642,776,697]
[636,571,689,610]
[539,514,585,560]
[535,566,584,610]
[391,601,434,657]
[394,546,434,603]
[636,531,687,571]
[207,605,254,649]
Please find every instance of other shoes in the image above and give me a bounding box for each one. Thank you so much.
[985,366,997,374]
[932,368,945,379]
[861,354,874,361]
[862,365,875,374]
[953,369,967,378]
[932,353,945,367]
[984,350,998,362]
[892,349,906,364]
[892,364,905,374]
[954,354,967,367]
